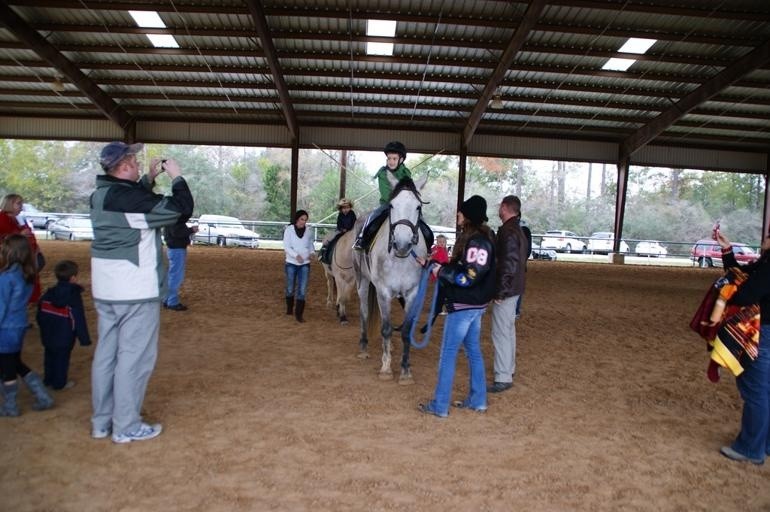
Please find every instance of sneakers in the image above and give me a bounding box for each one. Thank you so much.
[486,380,514,394]
[111,424,163,444]
[91,424,111,440]
[720,444,766,464]
[417,402,450,419]
[453,398,489,412]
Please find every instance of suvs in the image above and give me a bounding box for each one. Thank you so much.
[588,233,629,254]
[541,231,587,253]
[195,214,260,249]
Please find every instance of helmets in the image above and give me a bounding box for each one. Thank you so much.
[383,141,406,156]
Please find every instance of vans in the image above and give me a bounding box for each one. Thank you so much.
[690,239,761,268]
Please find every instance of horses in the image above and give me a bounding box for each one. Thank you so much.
[352,176,434,386]
[319,213,368,326]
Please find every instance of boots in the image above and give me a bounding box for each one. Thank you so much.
[285,295,294,316]
[295,298,306,324]
[1,380,21,419]
[23,370,54,411]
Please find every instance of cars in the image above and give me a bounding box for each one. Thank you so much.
[636,240,667,256]
[16,204,60,228]
[186,219,199,228]
[48,216,96,241]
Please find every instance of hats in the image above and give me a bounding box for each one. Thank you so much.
[460,195,488,222]
[336,199,352,207]
[100,143,144,171]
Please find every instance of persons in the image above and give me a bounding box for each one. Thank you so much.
[487,195,532,393]
[0,194,42,305]
[429,234,453,315]
[417,195,496,417]
[284,210,316,323]
[0,235,54,416]
[35,261,91,391]
[163,209,198,311]
[352,141,432,252]
[90,142,194,443]
[327,198,357,246]
[711,219,770,466]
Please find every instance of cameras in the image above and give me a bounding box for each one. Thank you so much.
[162,160,167,170]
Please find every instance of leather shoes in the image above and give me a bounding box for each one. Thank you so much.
[166,302,188,312]
